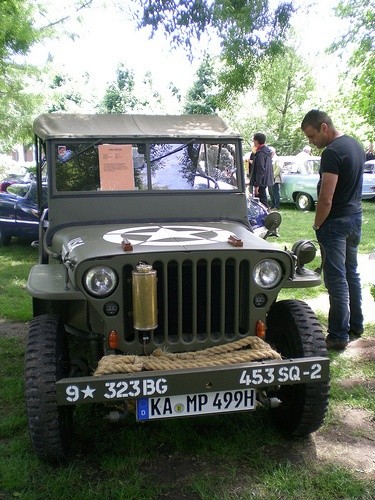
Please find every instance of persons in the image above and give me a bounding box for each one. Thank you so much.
[301,109,366,350]
[268,148,282,210]
[296,146,313,170]
[366,144,375,161]
[248,133,274,206]
[197,143,235,186]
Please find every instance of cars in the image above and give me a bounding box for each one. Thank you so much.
[264,155,322,212]
[360,159,375,199]
[0,157,48,247]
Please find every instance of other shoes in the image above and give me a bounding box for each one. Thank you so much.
[348,327,363,334]
[326,338,348,349]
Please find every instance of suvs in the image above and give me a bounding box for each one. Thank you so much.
[24,111,334,468]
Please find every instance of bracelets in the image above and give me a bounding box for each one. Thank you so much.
[312,225,319,230]
